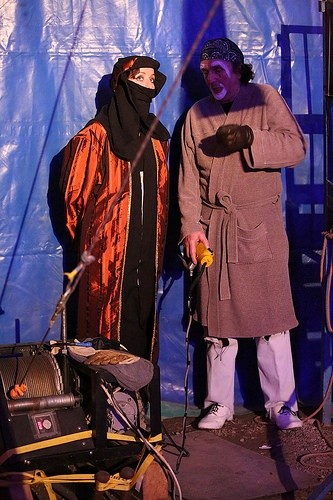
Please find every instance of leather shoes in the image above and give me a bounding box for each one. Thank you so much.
[198,403,233,429]
[271,404,302,429]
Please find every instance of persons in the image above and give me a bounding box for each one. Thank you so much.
[58,54,170,428]
[177,38,307,431]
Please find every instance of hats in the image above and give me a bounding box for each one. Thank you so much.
[110,56,166,97]
[200,38,244,63]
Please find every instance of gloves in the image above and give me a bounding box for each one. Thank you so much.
[216,124,253,154]
[84,351,136,366]
